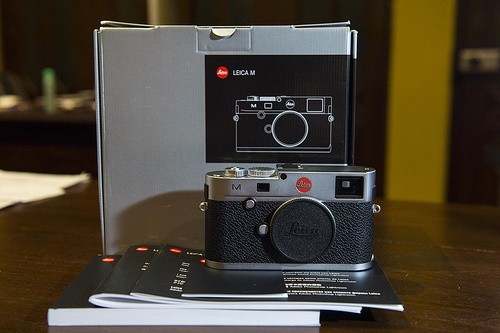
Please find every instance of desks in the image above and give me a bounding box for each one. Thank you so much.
[0,170,500,333]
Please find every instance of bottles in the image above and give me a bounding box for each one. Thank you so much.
[42,68,55,114]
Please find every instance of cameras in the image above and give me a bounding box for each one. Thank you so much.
[204,163,381,272]
[233,95,335,154]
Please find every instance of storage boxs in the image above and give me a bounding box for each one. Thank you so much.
[93,20,358,256]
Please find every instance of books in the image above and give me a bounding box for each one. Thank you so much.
[47,242,405,326]
